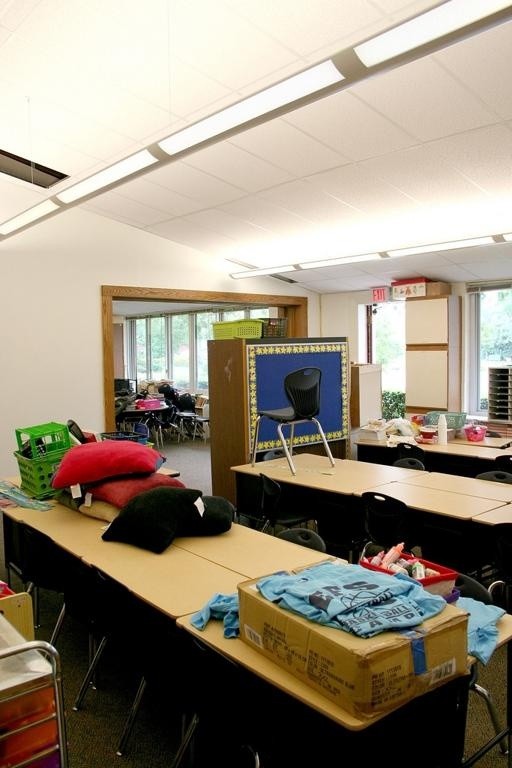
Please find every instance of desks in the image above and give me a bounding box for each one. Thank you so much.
[230,448,512,526]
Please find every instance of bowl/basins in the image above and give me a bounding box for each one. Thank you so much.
[419,430,436,439]
[464,424,487,441]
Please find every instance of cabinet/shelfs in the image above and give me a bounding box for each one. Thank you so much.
[207,337,351,508]
[405,295,460,413]
[351,364,382,428]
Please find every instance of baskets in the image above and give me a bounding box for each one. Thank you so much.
[212,317,288,339]
[14,420,84,501]
[426,412,466,429]
[465,425,487,442]
[100,431,154,448]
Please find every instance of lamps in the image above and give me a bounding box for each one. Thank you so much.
[0,0,512,244]
[229,231,511,280]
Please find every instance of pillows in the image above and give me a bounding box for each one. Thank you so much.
[49,439,237,553]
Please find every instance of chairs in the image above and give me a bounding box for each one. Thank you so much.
[251,366,335,475]
[114,379,209,449]
[353,430,512,473]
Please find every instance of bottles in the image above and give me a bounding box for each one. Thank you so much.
[437,414,448,446]
[362,541,441,580]
